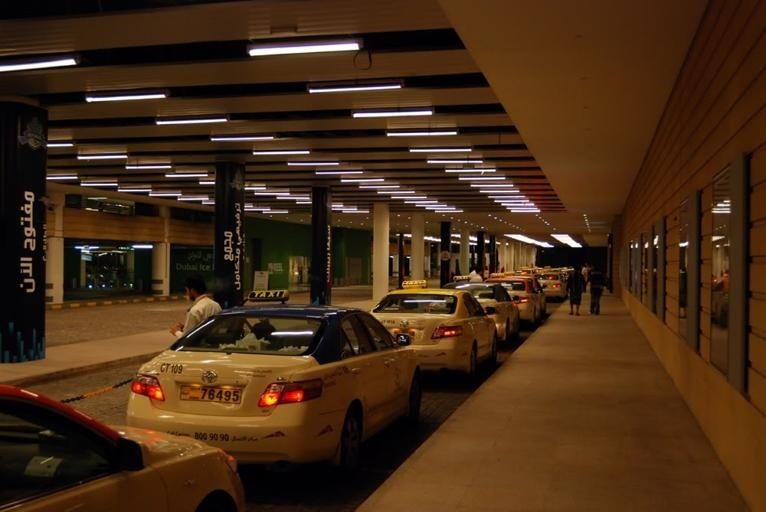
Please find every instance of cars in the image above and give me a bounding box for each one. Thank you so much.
[126,288,424,483]
[482,266,575,330]
[366,280,498,388]
[441,276,521,352]
[0,383,245,512]
[711,278,729,329]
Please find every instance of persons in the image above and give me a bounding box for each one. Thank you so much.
[170,275,222,340]
[589,264,607,315]
[679,266,688,318]
[566,266,586,315]
[581,263,592,285]
[470,267,483,283]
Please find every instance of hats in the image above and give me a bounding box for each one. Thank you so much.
[711,278,729,329]
[0,383,245,512]
